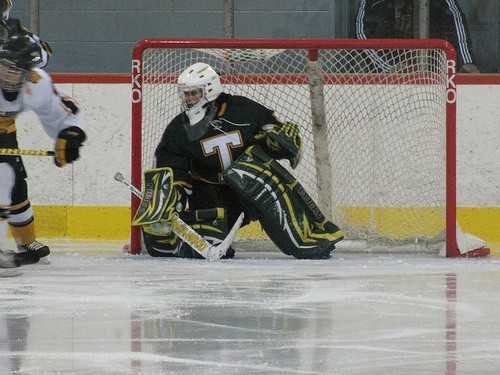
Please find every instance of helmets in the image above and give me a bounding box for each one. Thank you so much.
[0,36,42,71]
[177,62,222,126]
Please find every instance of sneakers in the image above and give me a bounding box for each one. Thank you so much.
[0,250,25,277]
[17,240,50,265]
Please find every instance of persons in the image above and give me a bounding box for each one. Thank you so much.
[139,62,344,259]
[0,0,87,270]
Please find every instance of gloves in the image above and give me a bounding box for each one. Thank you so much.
[54,126,86,167]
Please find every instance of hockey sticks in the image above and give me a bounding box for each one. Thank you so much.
[114,171,245,262]
[0,148,56,156]
